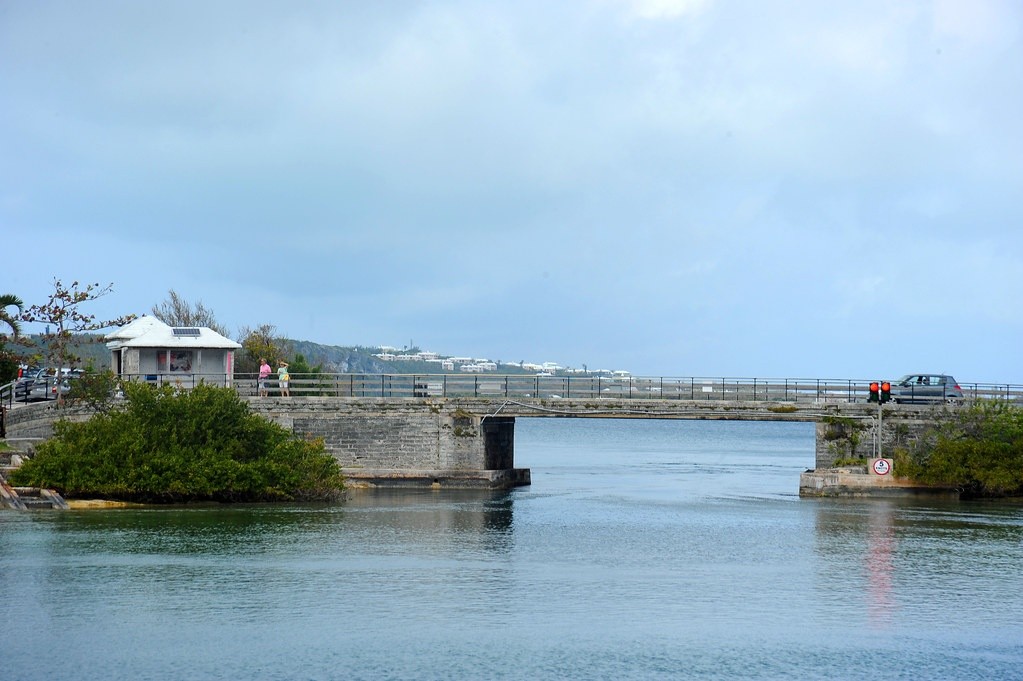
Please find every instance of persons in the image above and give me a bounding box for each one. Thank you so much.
[259,358,271,397]
[277,361,289,396]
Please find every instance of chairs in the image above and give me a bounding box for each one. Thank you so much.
[916,378,922,384]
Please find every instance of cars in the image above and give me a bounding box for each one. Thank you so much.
[10,364,43,395]
[867,373,965,412]
[29,366,88,398]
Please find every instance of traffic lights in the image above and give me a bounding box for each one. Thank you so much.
[869,382,879,402]
[880,382,890,402]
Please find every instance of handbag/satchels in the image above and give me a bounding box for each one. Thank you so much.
[282,373,290,381]
